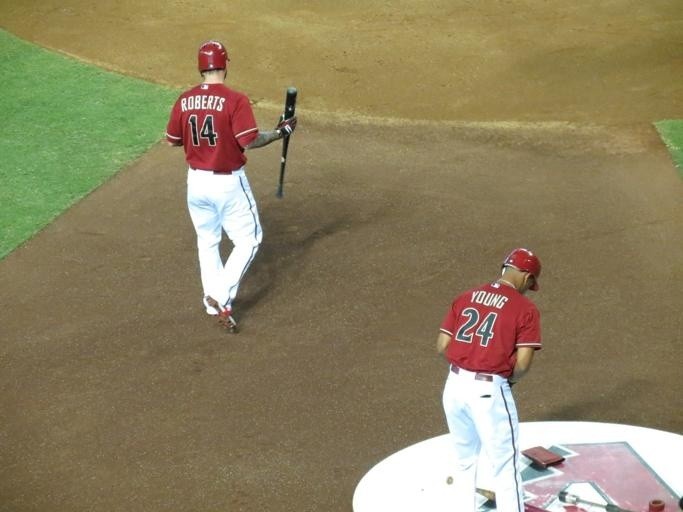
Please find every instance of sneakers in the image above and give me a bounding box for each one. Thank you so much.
[206,294,237,336]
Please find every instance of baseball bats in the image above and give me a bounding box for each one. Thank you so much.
[275,88,297,198]
[447,477,548,512]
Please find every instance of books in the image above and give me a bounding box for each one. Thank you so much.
[521,445,565,470]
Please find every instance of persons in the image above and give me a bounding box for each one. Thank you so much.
[433,246,542,512]
[164,39,296,337]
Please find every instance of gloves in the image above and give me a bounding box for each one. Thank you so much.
[276,114,297,138]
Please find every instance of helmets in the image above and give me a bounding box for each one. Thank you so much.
[503,247,542,292]
[198,40,230,72]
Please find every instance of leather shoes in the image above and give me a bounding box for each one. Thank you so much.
[451,366,493,383]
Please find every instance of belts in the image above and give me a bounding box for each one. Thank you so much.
[191,167,232,175]
